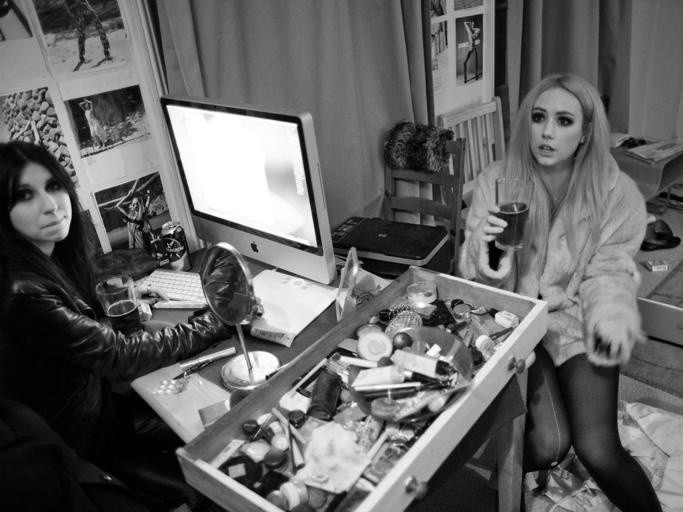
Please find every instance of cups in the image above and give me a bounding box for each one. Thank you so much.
[495,176,535,252]
[96,275,143,339]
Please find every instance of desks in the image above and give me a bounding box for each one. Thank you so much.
[100,248,547,512]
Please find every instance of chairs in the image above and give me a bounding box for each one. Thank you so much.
[366,94,509,276]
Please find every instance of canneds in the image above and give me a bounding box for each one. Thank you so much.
[160,221,192,271]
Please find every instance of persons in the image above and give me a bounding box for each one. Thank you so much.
[78,98,104,148]
[64,0,113,62]
[116,188,151,251]
[0,139,262,508]
[455,71,663,511]
[463,22,480,83]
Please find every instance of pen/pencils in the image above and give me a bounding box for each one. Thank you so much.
[172,347,236,380]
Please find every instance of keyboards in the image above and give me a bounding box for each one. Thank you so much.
[130,268,205,300]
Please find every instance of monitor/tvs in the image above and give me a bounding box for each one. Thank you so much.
[157,94,337,284]
